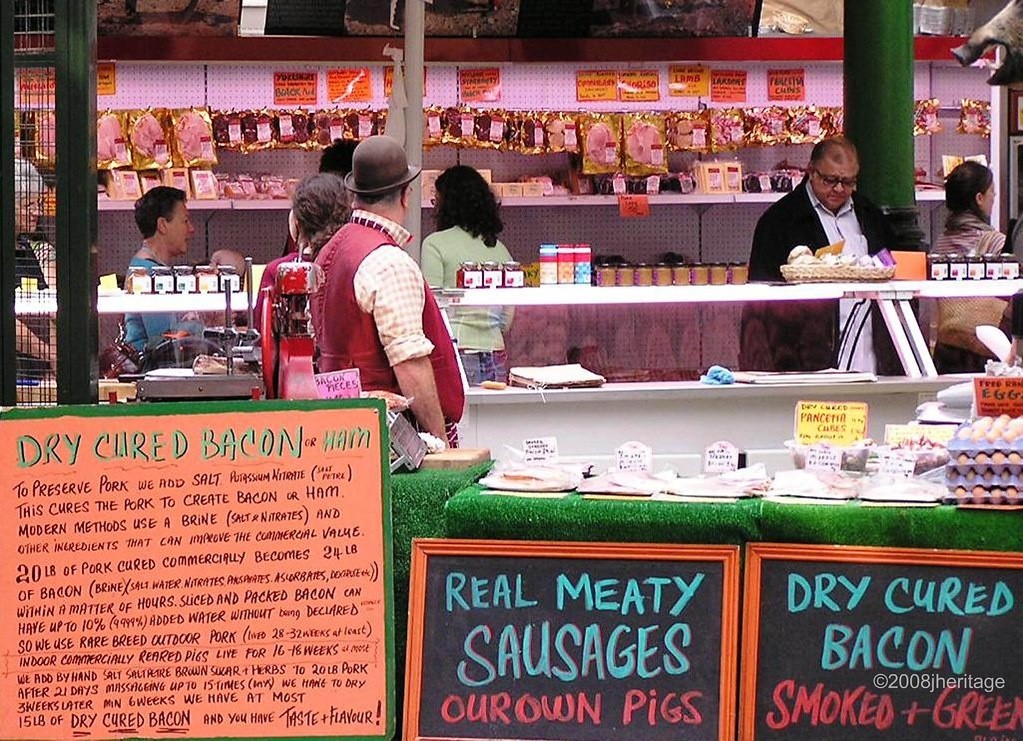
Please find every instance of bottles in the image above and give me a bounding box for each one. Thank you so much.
[539,242,558,285]
[574,242,591,283]
[558,243,574,283]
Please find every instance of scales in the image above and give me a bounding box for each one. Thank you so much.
[136,371,264,401]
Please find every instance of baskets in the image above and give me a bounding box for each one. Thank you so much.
[779,264,896,284]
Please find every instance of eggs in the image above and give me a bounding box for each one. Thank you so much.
[955,414,1023,497]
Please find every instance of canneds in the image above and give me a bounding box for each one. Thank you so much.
[594,261,749,287]
[925,252,1021,280]
[126,264,240,295]
[455,260,526,289]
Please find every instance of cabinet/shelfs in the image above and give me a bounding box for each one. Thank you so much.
[17,31,1007,399]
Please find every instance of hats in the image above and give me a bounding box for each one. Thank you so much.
[344,135,422,194]
[14,159,52,193]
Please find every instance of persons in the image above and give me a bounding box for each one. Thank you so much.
[738,137,919,376]
[122,135,516,450]
[926,161,1023,375]
[14,158,57,387]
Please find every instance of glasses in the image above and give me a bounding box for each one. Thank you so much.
[813,166,858,190]
[16,197,46,213]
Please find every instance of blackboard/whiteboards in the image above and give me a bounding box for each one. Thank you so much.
[742,541,1023,741]
[416,545,741,741]
